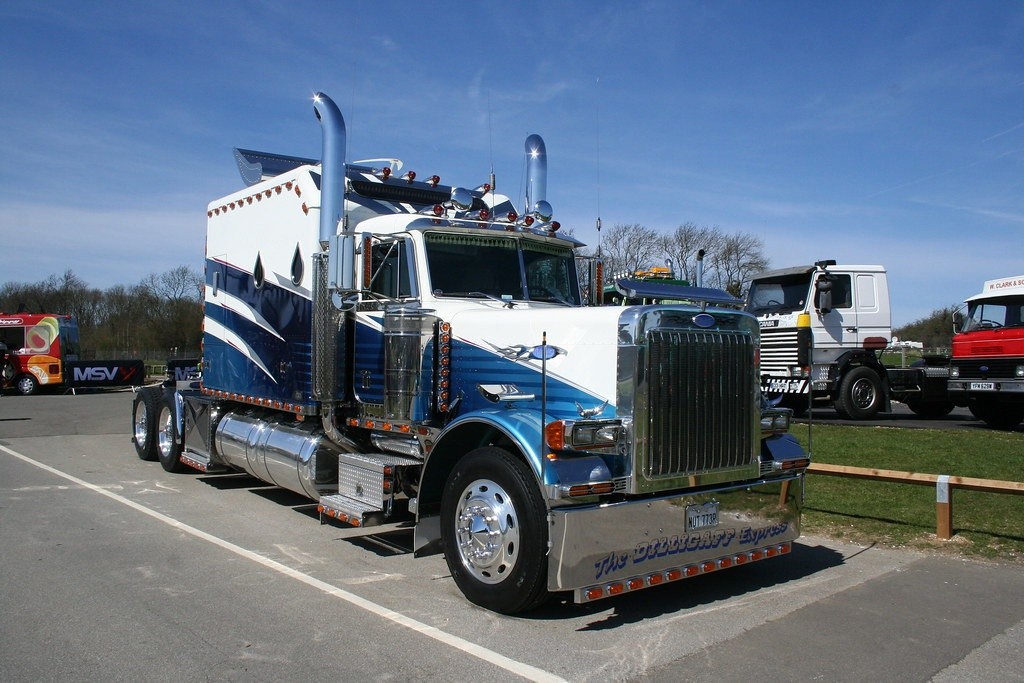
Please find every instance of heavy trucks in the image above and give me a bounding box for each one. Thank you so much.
[132,92,815,620]
[737,259,956,420]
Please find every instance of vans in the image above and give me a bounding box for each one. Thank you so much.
[0,312,82,396]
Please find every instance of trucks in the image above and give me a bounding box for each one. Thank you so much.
[947,275,1024,430]
